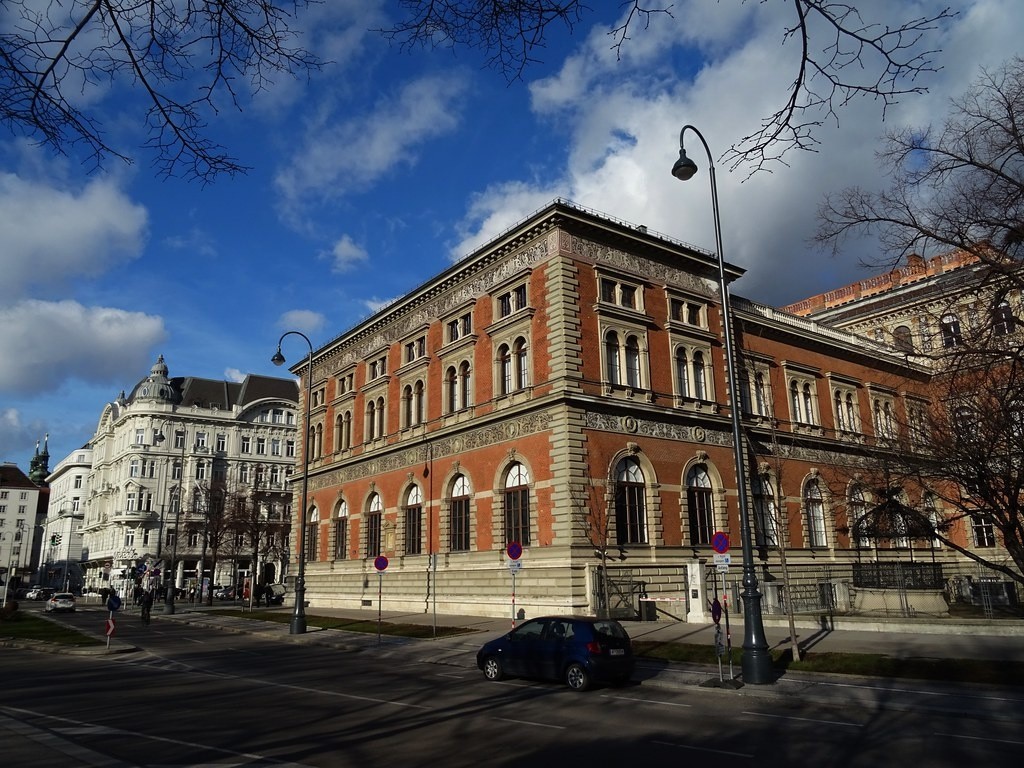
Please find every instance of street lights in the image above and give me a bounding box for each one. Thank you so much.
[35,524,48,585]
[2,531,14,608]
[20,524,30,583]
[156,417,186,615]
[672,126,775,684]
[58,501,74,593]
[271,332,313,634]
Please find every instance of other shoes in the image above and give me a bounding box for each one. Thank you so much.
[256,606,259,608]
[266,605,269,607]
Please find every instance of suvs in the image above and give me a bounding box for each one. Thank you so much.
[46,593,76,612]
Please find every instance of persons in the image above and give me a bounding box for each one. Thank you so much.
[254,582,275,608]
[244,581,250,601]
[130,581,197,624]
[83,585,116,606]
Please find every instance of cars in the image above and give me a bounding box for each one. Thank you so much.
[16,585,53,600]
[215,583,242,601]
[476,615,633,692]
